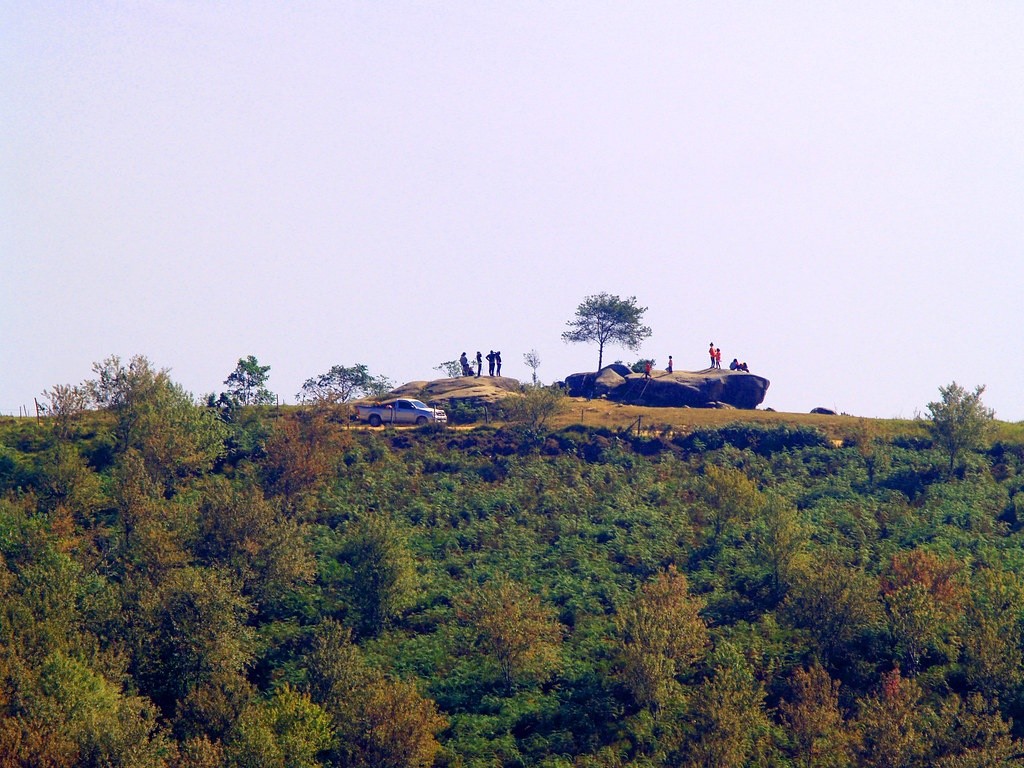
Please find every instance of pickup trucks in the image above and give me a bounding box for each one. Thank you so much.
[357,397,447,428]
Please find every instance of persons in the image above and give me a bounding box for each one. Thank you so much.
[459,350,502,377]
[729,359,750,373]
[709,342,722,370]
[668,355,673,373]
[645,360,651,379]
